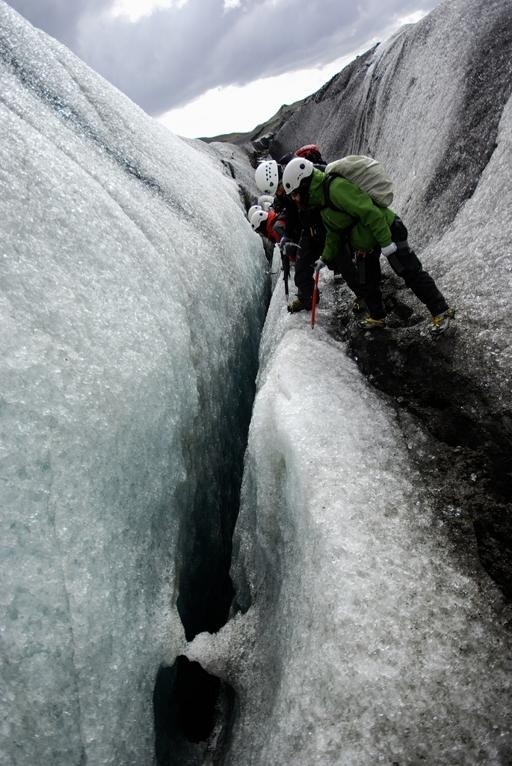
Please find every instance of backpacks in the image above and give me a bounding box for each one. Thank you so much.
[323,154,393,212]
[294,145,321,160]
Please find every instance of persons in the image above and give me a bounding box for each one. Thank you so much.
[247,144,450,327]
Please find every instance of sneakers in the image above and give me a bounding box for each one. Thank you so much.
[430,308,451,335]
[353,316,386,331]
[287,294,319,313]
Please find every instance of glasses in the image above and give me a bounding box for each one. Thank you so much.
[287,182,306,199]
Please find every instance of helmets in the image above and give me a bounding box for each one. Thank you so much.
[248,205,262,223]
[255,160,278,196]
[250,211,269,232]
[258,196,275,210]
[282,157,313,195]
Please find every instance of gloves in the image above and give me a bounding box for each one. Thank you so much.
[381,242,397,257]
[278,237,295,251]
[312,255,325,271]
